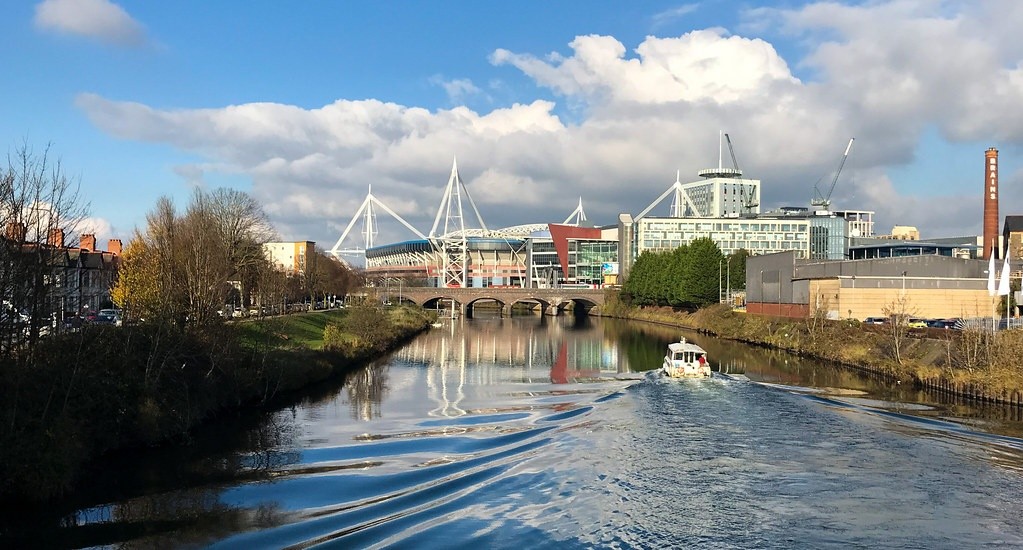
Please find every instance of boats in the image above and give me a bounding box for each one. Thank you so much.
[663,336,711,381]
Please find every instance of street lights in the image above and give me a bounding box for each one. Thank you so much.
[851,276,857,318]
[901,271,908,327]
[400,278,406,305]
[719,260,723,305]
[727,259,730,305]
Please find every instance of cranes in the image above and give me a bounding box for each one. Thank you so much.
[724,134,761,219]
[809,138,855,211]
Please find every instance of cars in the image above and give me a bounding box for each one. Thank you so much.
[863,316,890,326]
[905,317,964,330]
[999,318,1021,330]
[0,300,122,353]
[185,299,343,323]
[845,318,862,322]
